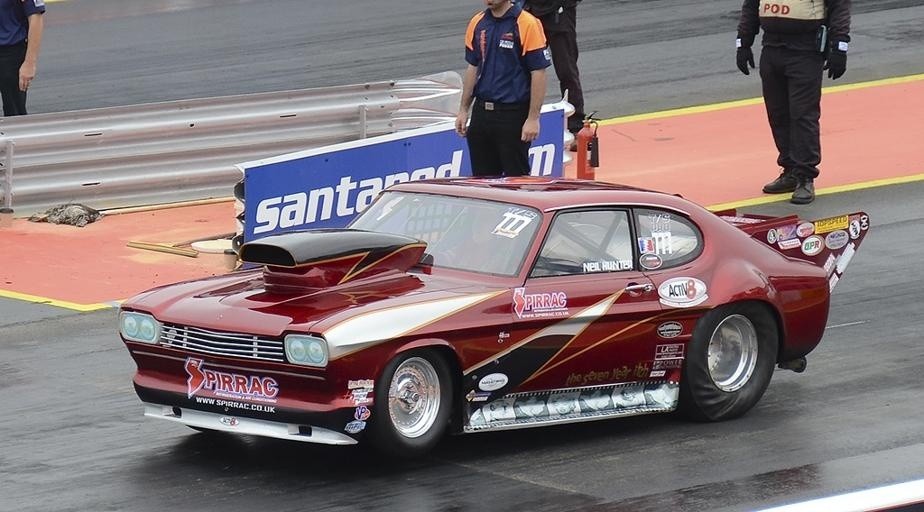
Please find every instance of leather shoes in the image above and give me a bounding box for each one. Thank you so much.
[765,170,817,204]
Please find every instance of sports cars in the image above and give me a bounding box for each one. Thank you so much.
[118,176,869,469]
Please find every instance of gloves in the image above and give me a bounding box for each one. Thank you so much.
[734,38,758,75]
[823,39,848,79]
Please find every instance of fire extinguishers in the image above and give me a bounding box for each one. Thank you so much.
[576,111,602,181]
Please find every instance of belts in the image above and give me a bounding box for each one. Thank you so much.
[471,98,528,113]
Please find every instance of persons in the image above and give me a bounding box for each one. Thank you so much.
[0,1,45,116]
[734,0,852,206]
[454,0,553,176]
[520,0,589,152]
